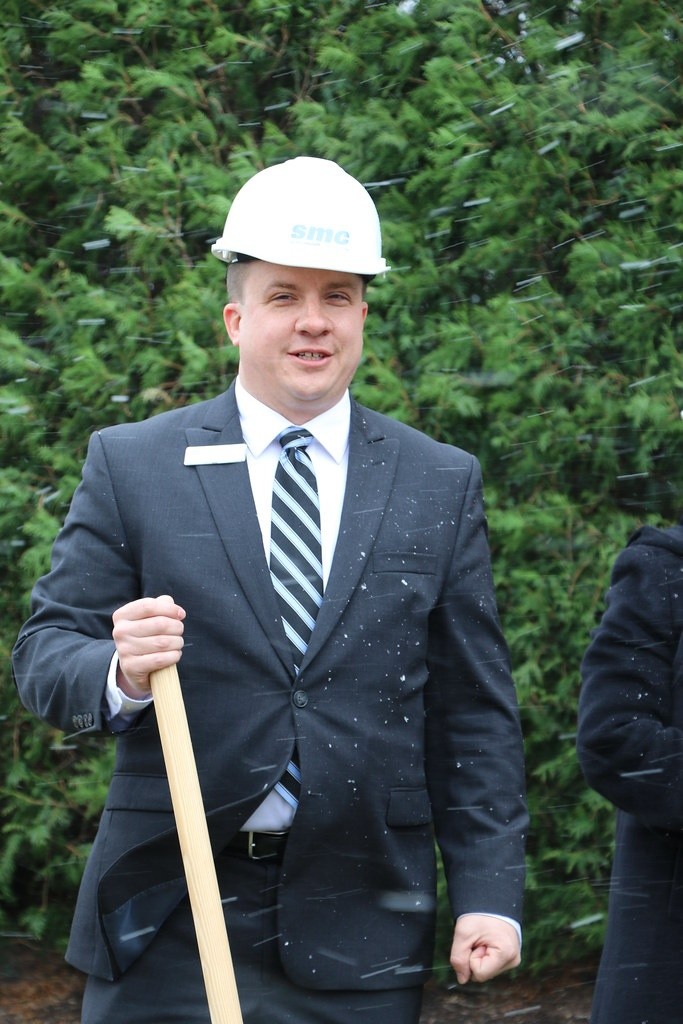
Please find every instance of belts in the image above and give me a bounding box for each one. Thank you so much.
[219,830,290,865]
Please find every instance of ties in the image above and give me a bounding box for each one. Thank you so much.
[269,426,324,813]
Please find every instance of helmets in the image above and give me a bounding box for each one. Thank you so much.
[211,156,391,275]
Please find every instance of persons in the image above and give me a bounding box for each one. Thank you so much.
[9,157,523,1024]
[575,525,683,1024]
